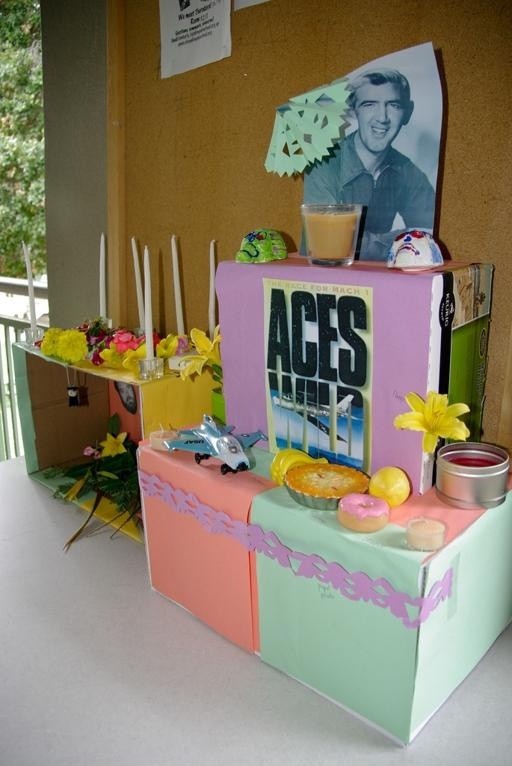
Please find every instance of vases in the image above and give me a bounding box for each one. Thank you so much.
[211,387,225,423]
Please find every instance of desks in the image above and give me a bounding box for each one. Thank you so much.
[13,338,215,545]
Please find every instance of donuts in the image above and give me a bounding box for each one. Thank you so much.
[335,492,389,533]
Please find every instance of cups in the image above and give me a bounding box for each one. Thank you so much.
[302,202,365,266]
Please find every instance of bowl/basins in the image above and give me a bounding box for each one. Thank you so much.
[406,518,447,550]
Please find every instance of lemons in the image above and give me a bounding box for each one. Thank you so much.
[369,466,411,508]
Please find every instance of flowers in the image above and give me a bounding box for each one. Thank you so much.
[394,390,473,455]
[176,323,223,396]
[82,411,143,511]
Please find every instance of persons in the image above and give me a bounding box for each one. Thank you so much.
[298,65,436,260]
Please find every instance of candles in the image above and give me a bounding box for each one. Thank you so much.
[98,233,107,325]
[406,514,448,553]
[208,238,219,337]
[143,245,157,372]
[20,238,39,341]
[148,421,180,455]
[131,236,145,335]
[170,235,187,334]
